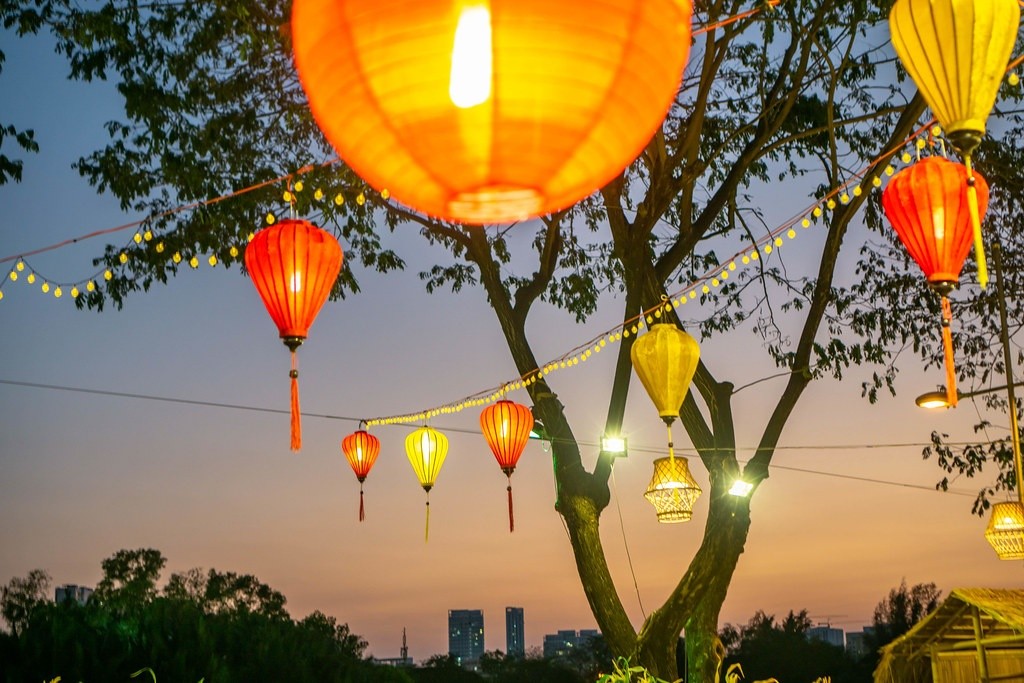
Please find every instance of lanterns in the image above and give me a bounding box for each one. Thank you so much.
[880,154,992,411]
[243,217,344,451]
[479,398,535,533]
[888,0,1023,291]
[631,322,702,517]
[289,0,696,228]
[340,430,380,523]
[403,428,451,542]
[985,499,1024,562]
[643,455,702,524]
